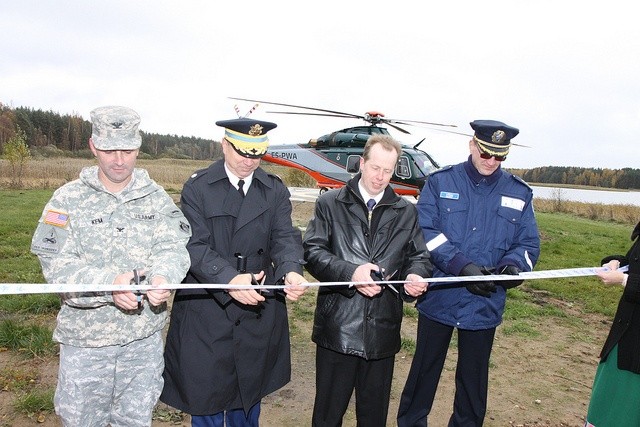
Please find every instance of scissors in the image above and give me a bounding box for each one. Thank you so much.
[363,263,400,296]
[479,264,512,287]
[125,271,153,310]
[242,273,270,302]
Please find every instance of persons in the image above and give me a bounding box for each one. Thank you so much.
[397,121,541,427]
[159,119,308,427]
[31,106,193,427]
[585,220,640,425]
[302,135,434,427]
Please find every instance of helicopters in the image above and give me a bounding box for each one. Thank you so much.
[227,92,532,205]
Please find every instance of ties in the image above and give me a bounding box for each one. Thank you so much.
[367,199,377,230]
[237,179,247,199]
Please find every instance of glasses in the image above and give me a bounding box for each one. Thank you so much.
[473,141,508,163]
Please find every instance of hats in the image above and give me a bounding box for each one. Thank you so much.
[215,117,278,161]
[89,105,144,151]
[469,119,521,159]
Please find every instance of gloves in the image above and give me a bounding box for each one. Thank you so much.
[498,266,526,291]
[461,262,499,300]
